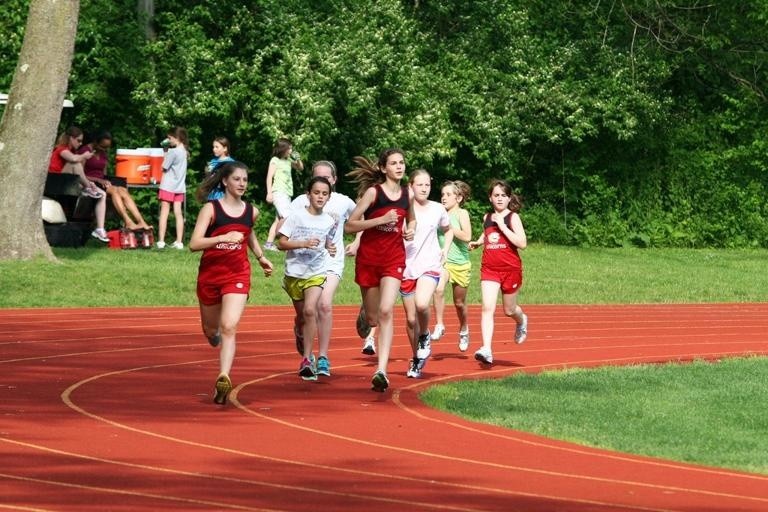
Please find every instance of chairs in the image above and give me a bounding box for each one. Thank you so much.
[40,172,127,248]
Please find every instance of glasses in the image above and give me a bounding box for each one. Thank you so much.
[77,139,84,143]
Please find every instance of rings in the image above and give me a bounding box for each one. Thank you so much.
[391,212,394,215]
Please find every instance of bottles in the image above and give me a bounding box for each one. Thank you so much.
[119,228,130,249]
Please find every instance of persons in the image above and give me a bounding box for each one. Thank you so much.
[467,180,528,366]
[360,326,377,356]
[205,136,236,202]
[430,180,472,352]
[263,138,304,252]
[398,167,455,378]
[344,149,417,393]
[48,125,111,242]
[79,129,154,231]
[274,159,364,381]
[156,126,190,249]
[277,176,339,377]
[189,160,273,406]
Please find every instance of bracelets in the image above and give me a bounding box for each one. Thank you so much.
[257,255,264,261]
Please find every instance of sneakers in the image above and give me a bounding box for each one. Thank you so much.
[213,374,233,405]
[513,314,528,345]
[294,326,331,383]
[208,331,221,348]
[91,228,185,252]
[263,243,280,253]
[356,303,494,393]
[80,187,103,199]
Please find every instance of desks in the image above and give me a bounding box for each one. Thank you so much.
[127,183,186,225]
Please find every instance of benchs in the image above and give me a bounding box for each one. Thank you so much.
[63,194,65,195]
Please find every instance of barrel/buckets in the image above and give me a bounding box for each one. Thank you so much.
[149,148,167,183]
[116,148,151,186]
